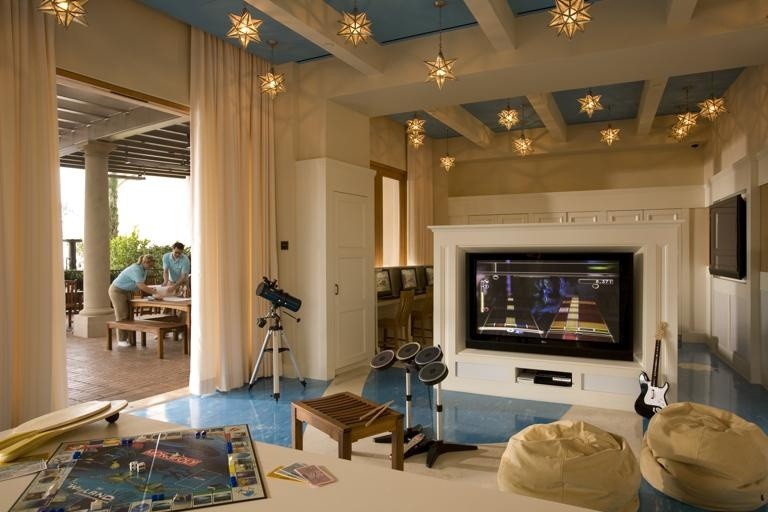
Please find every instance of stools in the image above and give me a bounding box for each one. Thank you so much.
[290,391,405,470]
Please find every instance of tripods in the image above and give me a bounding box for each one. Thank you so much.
[248,327,306,402]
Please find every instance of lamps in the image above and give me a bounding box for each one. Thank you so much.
[336,3,374,47]
[36,0,89,30]
[599,105,622,148]
[406,112,425,135]
[511,105,536,159]
[225,8,263,50]
[669,89,699,146]
[576,86,604,119]
[546,1,593,41]
[422,7,458,90]
[256,45,288,100]
[440,128,456,171]
[408,135,424,149]
[697,76,729,121]
[497,98,519,132]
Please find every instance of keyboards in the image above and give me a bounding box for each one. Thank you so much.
[379,295,399,301]
[413,290,426,295]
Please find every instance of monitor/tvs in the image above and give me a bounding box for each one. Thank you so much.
[400,268,419,289]
[375,269,393,297]
[425,266,433,286]
[709,194,746,279]
[465,252,634,362]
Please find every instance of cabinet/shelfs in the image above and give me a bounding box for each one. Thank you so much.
[455,356,645,396]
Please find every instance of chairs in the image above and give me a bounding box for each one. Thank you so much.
[411,286,433,345]
[65,278,79,327]
[379,289,415,350]
[135,289,152,316]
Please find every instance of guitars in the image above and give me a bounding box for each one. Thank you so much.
[635,322,667,419]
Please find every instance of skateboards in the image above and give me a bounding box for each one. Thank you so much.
[0,399,129,463]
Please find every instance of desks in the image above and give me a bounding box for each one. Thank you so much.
[0,411,604,512]
[66,286,83,313]
[127,297,190,354]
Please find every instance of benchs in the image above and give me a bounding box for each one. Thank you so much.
[105,319,187,359]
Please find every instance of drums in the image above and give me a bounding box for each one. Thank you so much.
[414,347,443,371]
[419,360,449,385]
[395,342,420,363]
[369,350,394,370]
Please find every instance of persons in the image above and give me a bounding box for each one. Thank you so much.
[154,243,190,341]
[108,254,163,347]
